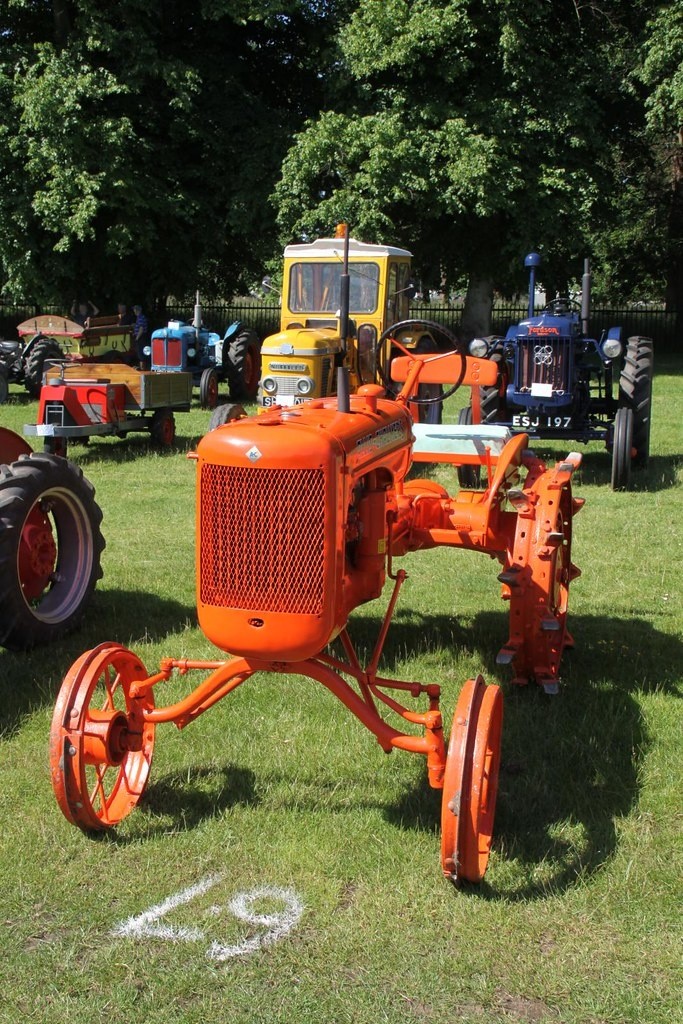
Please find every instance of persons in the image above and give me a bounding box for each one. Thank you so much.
[70,299,100,329]
[208,271,273,304]
[114,301,148,371]
